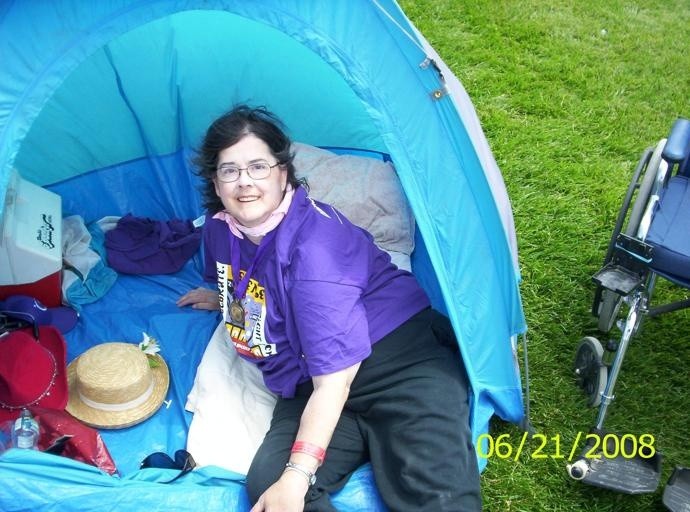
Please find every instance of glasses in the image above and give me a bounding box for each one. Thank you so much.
[128,449,197,484]
[212,162,283,183]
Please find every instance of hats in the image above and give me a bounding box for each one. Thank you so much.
[1,296,79,336]
[0,325,68,424]
[64,342,170,431]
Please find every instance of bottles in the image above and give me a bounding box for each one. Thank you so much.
[10,410,39,451]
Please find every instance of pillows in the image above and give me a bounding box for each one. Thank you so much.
[287,142,416,257]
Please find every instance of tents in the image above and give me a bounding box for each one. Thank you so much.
[0,0,528,512]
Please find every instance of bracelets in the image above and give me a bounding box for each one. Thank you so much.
[290,439,328,466]
[281,460,319,488]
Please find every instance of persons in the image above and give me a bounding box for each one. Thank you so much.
[175,101,483,512]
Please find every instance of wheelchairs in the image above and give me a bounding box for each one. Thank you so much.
[564,117,690,512]
